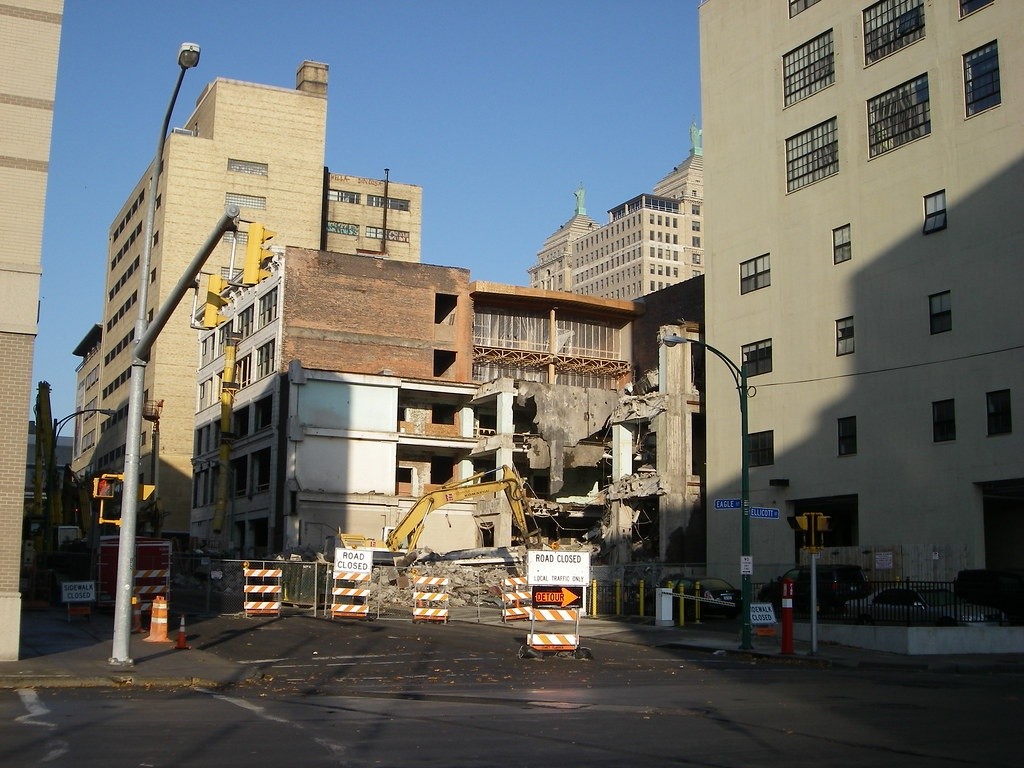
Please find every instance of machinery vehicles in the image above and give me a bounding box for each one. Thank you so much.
[24,380,175,610]
[325,462,547,568]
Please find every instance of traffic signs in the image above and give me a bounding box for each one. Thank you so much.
[714,500,742,511]
[750,507,779,520]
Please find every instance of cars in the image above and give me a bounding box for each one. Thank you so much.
[628,573,743,620]
[834,587,1010,628]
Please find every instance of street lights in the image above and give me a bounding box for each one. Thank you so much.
[106,40,200,670]
[42,408,117,541]
[662,332,757,650]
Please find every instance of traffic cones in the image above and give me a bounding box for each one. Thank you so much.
[142,596,173,642]
[130,602,147,633]
[172,613,192,649]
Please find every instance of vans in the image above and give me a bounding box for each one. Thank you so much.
[758,563,869,621]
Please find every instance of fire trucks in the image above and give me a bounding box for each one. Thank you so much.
[98,535,174,612]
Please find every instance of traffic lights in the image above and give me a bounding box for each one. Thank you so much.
[139,483,155,501]
[92,476,115,499]
[787,513,807,531]
[817,516,831,531]
